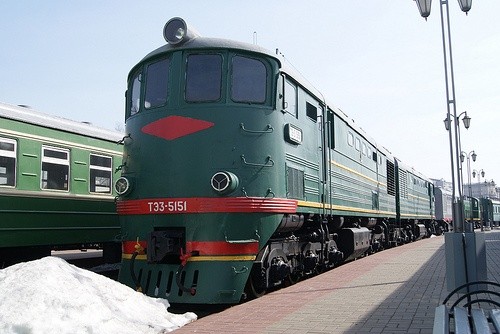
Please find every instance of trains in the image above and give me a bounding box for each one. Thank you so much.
[114,16,500,303]
[1,102,125,269]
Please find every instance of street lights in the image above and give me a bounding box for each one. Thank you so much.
[443,111,471,199]
[473,169,485,198]
[460,151,477,198]
[484,179,495,199]
[411,0,488,293]
